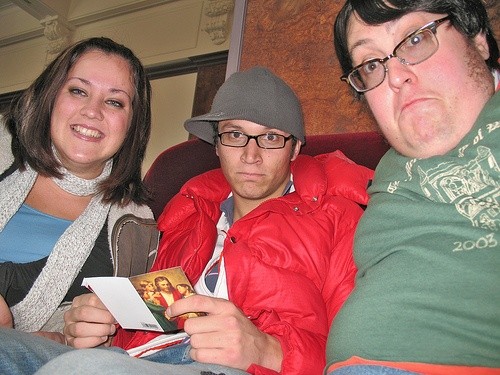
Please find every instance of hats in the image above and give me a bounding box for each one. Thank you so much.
[183,66,306,144]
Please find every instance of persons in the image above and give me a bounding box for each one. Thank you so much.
[323,0,499,375]
[0,36,160,353]
[0,67,376,375]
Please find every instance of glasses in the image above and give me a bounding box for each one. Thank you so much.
[217,130,297,149]
[340,15,454,94]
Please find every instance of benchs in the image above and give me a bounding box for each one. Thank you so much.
[142,131,391,243]
